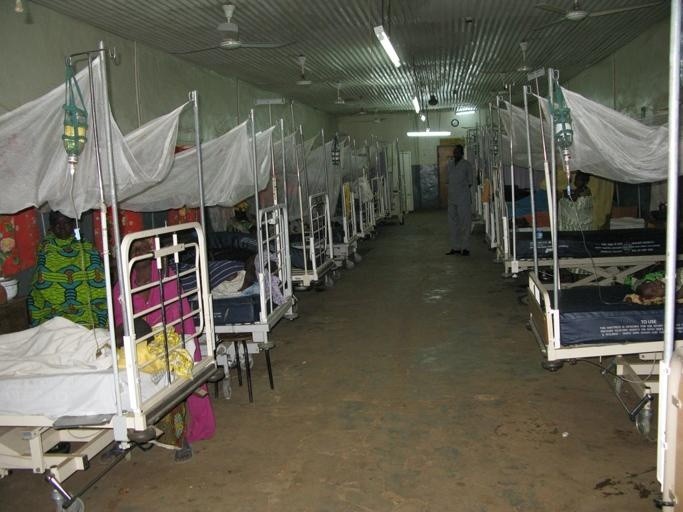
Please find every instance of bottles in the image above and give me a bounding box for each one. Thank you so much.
[331,147,340,166]
[553,107,573,149]
[488,133,497,153]
[63,106,88,165]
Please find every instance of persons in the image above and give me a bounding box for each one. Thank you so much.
[112,235,215,440]
[563,171,592,203]
[511,217,529,228]
[635,278,683,299]
[27,206,110,330]
[106,319,156,349]
[446,144,473,255]
[211,250,278,300]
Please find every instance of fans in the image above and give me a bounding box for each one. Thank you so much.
[167,5,297,54]
[256,55,338,88]
[532,0,663,31]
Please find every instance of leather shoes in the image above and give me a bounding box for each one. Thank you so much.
[446,249,461,255]
[463,249,470,256]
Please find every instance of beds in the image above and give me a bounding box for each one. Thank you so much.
[0,173,393,510]
[471,184,683,371]
